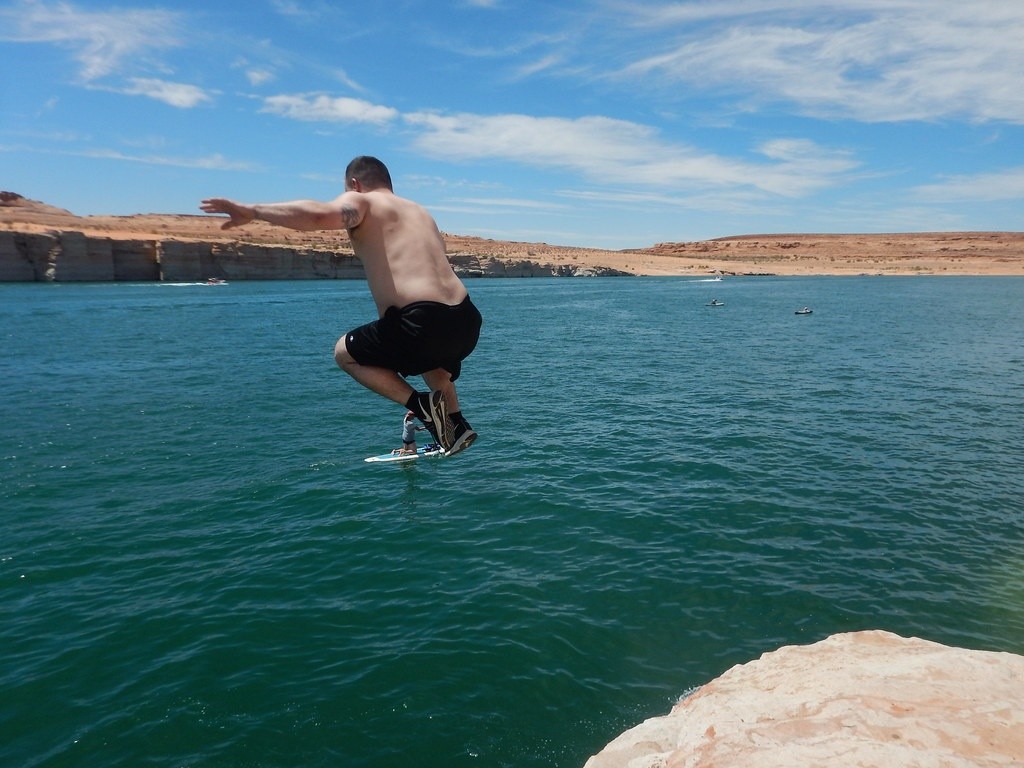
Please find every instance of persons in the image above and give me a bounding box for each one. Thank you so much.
[199,155,483,456]
[391,411,426,457]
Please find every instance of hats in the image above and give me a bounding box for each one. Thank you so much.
[406,410,415,418]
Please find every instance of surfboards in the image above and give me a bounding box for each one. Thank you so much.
[705,303,724,306]
[364,444,446,463]
[795,311,813,314]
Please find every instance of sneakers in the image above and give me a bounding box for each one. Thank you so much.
[417,389,454,451]
[444,417,478,457]
